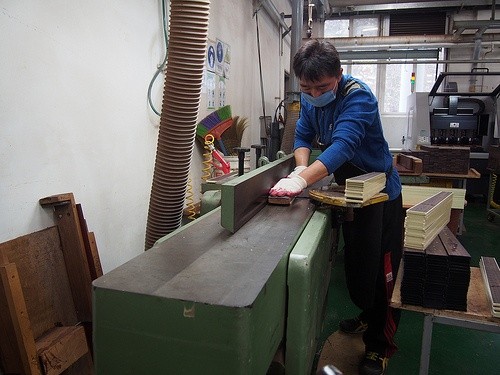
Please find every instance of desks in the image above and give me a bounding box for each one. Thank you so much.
[401,184,466,237]
[390,255,500,375]
[398,167,481,235]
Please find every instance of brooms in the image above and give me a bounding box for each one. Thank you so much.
[196,105,249,156]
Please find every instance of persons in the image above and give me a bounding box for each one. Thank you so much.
[267,38,406,375]
